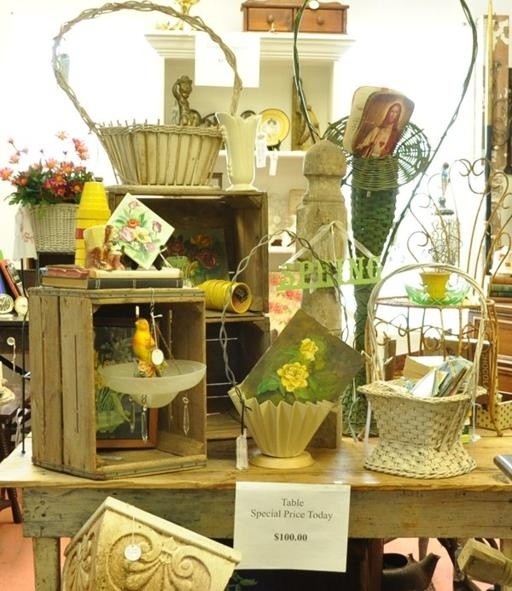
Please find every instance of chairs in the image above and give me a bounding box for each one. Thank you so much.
[360,152,512,445]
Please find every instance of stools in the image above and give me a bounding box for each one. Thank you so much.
[1,379,32,527]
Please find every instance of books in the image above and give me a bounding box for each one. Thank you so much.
[486,274,512,304]
[41,260,184,289]
[0,260,29,297]
[398,354,473,397]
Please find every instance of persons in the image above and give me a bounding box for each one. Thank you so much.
[356,103,400,158]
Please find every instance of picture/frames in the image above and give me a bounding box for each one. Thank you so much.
[205,170,225,188]
[150,213,238,287]
[89,311,164,449]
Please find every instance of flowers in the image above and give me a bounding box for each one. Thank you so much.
[0,128,104,209]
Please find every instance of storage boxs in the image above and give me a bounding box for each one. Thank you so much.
[25,283,211,480]
[107,188,272,317]
[201,313,273,442]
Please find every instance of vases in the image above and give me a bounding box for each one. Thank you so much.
[25,201,79,265]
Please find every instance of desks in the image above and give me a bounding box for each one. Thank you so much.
[0,312,29,356]
[0,425,511,591]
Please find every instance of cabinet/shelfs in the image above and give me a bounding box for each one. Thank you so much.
[208,146,310,332]
[238,0,353,33]
[465,299,512,397]
